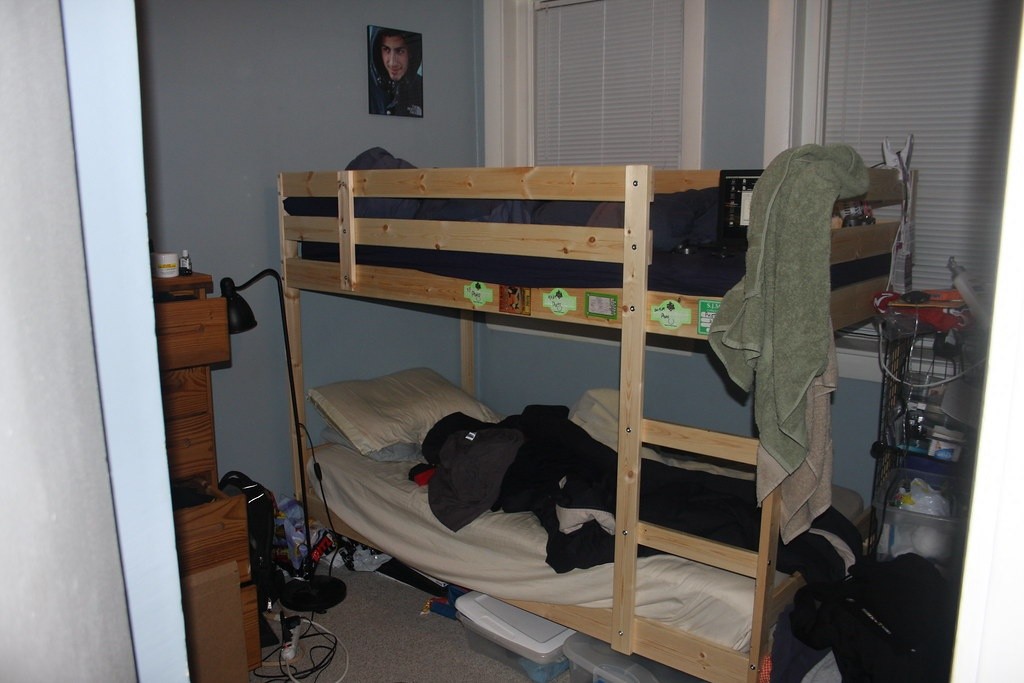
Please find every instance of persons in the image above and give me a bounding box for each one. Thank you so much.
[367,26,422,117]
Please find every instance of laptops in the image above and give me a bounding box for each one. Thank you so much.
[695,169,765,259]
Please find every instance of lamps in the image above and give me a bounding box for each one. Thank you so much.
[219,269,345,612]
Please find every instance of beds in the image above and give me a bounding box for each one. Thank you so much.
[272,164,909,683]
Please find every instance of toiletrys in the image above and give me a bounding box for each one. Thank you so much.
[946,252,992,327]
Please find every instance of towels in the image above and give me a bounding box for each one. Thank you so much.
[705,138,872,548]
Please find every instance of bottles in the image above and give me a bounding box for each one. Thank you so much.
[179,250,192,276]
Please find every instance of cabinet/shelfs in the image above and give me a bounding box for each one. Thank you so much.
[865,290,984,567]
[148,266,263,671]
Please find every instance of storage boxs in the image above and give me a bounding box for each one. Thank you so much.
[562,633,712,683]
[454,592,577,683]
[877,484,960,577]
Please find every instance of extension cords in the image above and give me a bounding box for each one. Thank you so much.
[281,616,302,660]
